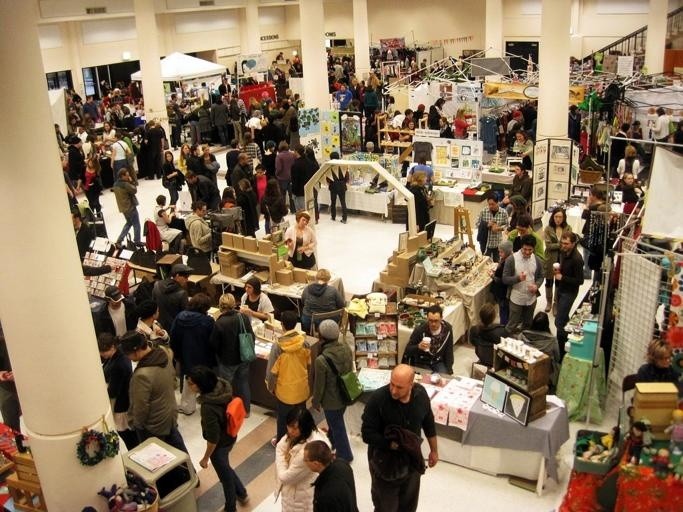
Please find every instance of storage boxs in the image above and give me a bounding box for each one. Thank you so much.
[12,451,40,484]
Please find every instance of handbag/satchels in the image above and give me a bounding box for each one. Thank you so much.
[186,248,211,275]
[337,371,363,406]
[129,247,168,269]
[239,333,257,362]
[126,232,134,250]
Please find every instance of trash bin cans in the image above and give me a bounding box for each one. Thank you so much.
[122,436,198,512]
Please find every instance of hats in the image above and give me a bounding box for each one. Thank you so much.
[319,319,338,339]
[171,265,193,277]
[118,330,145,353]
[105,286,125,304]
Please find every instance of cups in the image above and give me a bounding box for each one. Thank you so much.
[422,337,431,352]
[552,262,560,272]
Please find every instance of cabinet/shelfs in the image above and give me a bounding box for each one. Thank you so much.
[375,113,428,157]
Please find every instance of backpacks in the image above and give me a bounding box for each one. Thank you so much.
[225,397,246,437]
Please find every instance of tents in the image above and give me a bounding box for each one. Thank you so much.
[131,51,229,106]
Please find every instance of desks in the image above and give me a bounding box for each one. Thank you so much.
[74,227,570,497]
[2,469,49,511]
[482,164,531,188]
[430,183,492,229]
[316,181,396,223]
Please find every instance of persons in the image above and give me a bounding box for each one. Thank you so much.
[569,104,670,271]
[325,45,444,121]
[269,49,303,79]
[0,332,24,435]
[360,97,538,234]
[639,337,683,399]
[88,263,453,512]
[467,190,585,394]
[326,152,351,224]
[51,77,321,263]
